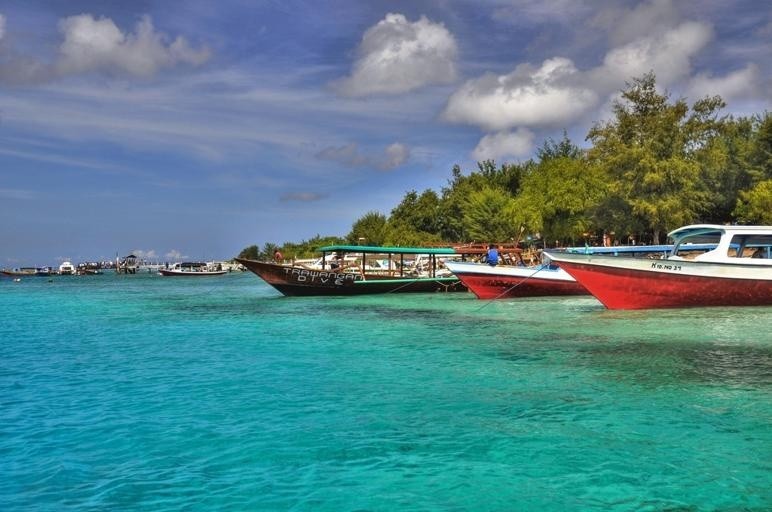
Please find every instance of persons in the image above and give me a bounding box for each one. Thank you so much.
[473,244,498,266]
[272,249,283,263]
